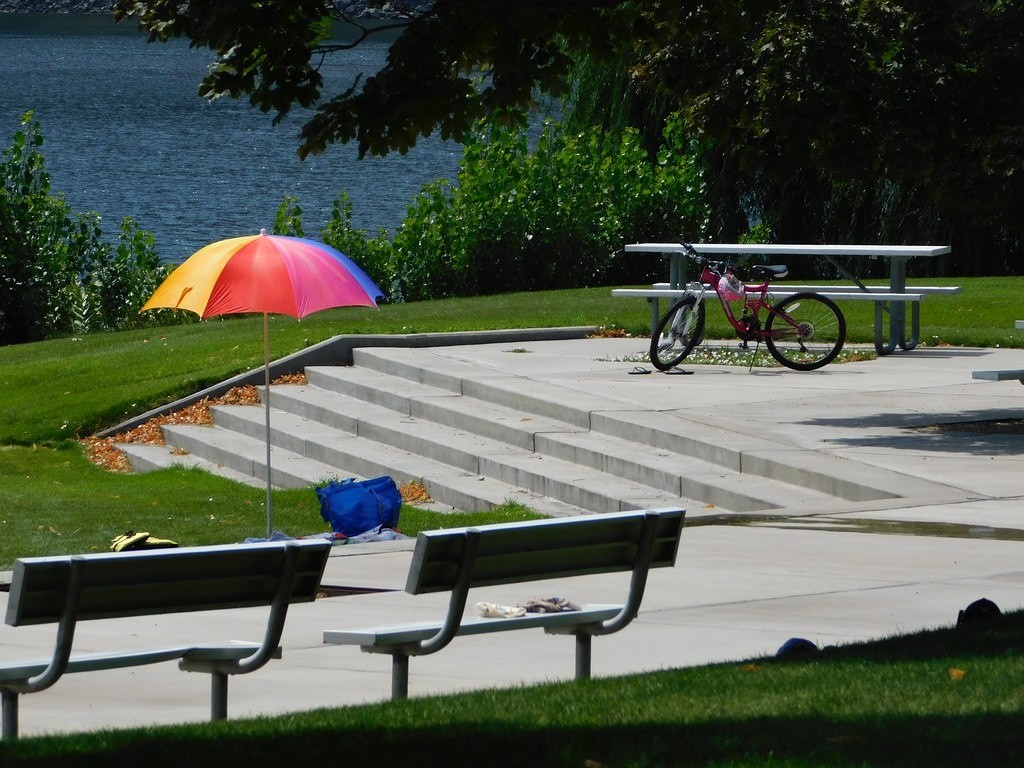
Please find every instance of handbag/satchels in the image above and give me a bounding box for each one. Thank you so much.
[315,476,402,536]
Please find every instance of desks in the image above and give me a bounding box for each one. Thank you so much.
[625,243,951,357]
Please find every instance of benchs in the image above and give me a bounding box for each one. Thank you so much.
[653,283,961,295]
[610,288,927,301]
[324,506,685,701]
[0,539,332,744]
[973,368,1024,382]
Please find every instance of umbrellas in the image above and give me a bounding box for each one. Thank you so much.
[138,227,384,540]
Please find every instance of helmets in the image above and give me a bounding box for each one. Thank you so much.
[717,269,745,302]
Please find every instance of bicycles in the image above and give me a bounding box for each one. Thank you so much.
[649,238,846,370]
